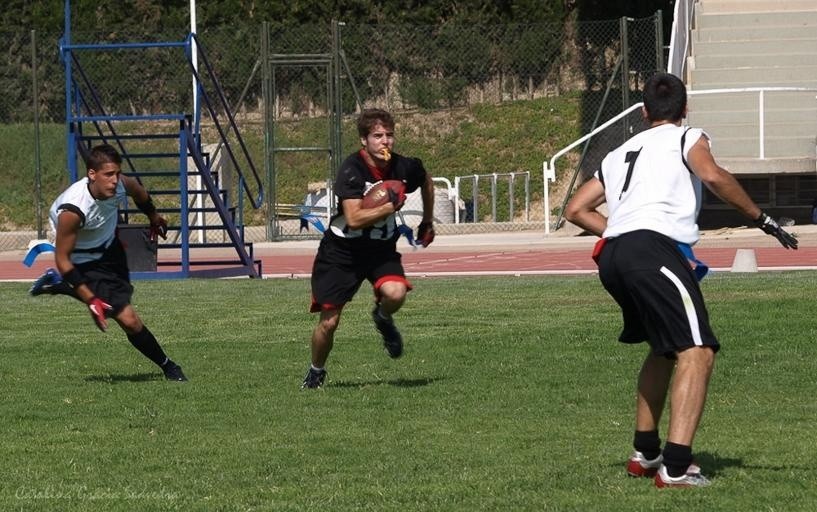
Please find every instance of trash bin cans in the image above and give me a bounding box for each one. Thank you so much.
[117,223,158,272]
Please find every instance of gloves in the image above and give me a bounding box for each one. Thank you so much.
[87,295,115,333]
[384,182,409,212]
[148,216,168,244]
[754,211,799,251]
[415,222,436,250]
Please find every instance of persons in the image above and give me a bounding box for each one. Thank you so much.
[301,108,436,389]
[564,73,798,488]
[29,142,188,381]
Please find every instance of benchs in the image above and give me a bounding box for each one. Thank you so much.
[64,111,264,282]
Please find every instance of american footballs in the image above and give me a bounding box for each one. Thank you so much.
[361,180,406,209]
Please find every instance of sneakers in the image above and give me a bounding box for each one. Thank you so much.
[29,266,63,296]
[161,360,190,383]
[372,306,404,359]
[625,449,675,480]
[653,461,714,491]
[298,368,329,390]
